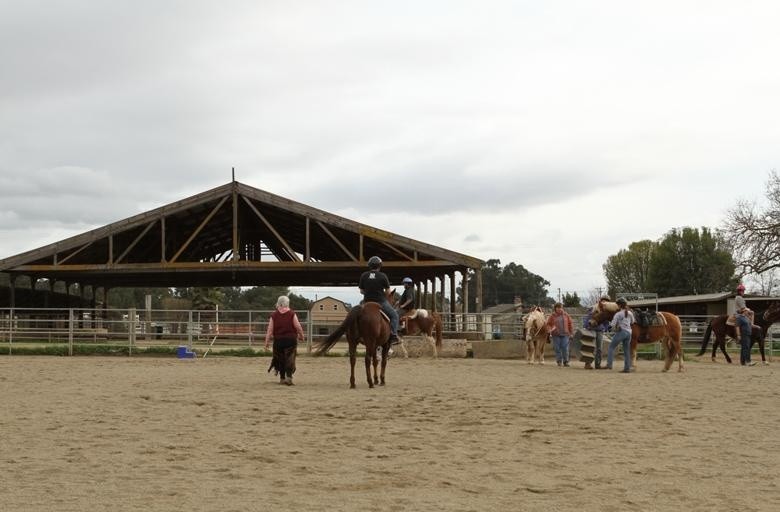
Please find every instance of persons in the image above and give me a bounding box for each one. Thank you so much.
[735,309,757,366]
[393,278,416,329]
[264,296,304,385]
[582,296,613,369]
[604,296,634,373]
[734,284,761,329]
[547,302,574,367]
[359,256,400,345]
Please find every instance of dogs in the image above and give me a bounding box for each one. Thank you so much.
[311,287,397,388]
[763,301,780,322]
[587,298,685,372]
[695,311,773,366]
[387,308,442,359]
[521,306,549,364]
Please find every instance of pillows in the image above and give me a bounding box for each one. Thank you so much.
[368,255,383,267]
[601,295,611,301]
[403,277,412,284]
[737,284,745,291]
[616,297,626,305]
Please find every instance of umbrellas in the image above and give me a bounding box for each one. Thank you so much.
[564,362,570,367]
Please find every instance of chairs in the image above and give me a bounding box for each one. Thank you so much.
[585,364,593,369]
[748,361,757,366]
[280,379,287,384]
[286,378,294,386]
[392,336,404,345]
